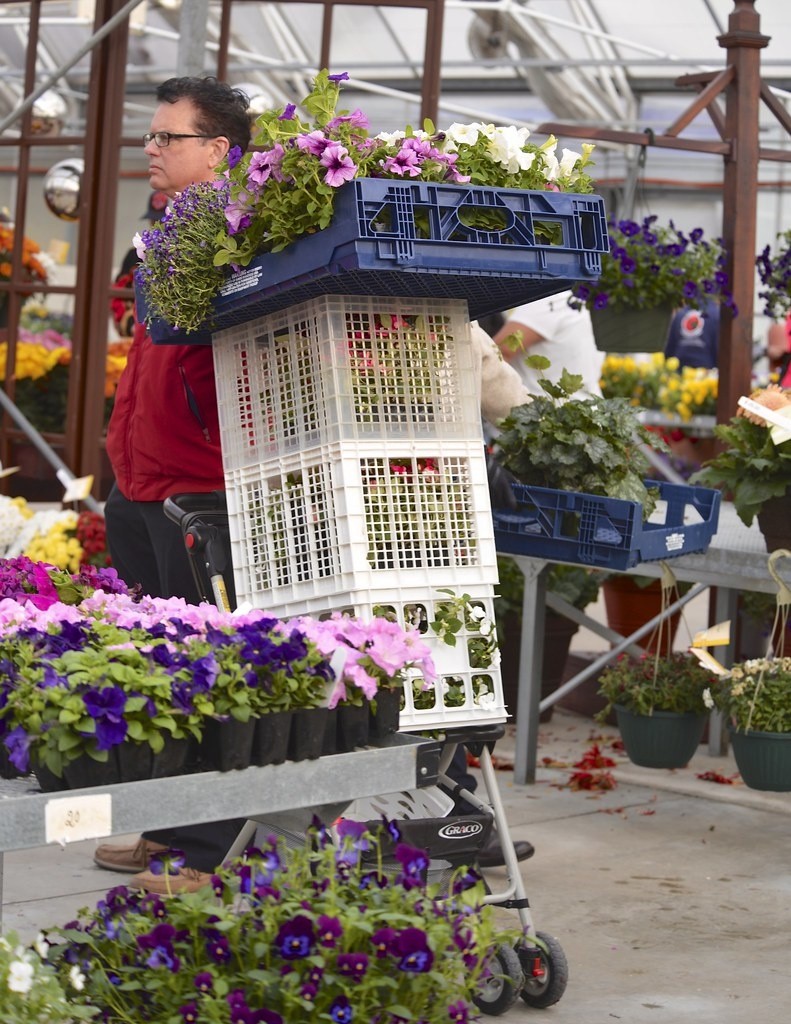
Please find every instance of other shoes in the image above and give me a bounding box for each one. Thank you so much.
[127,863,212,894]
[92,838,173,873]
[479,831,533,866]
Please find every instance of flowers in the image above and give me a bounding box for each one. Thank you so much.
[0,494,438,777]
[0,814,551,1024]
[593,650,791,737]
[0,72,791,425]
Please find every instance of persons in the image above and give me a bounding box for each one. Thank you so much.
[495,289,604,408]
[767,308,791,391]
[94,76,258,895]
[111,191,170,338]
[430,319,537,866]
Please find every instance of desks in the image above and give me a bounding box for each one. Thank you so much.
[0,733,438,932]
[497,500,791,785]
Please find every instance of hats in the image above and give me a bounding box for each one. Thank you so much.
[141,190,173,221]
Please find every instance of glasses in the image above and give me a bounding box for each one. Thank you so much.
[143,131,220,148]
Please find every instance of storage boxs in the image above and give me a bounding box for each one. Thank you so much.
[211,298,482,473]
[131,177,613,346]
[254,586,515,731]
[224,440,501,621]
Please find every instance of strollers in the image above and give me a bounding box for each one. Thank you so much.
[162,485,568,1011]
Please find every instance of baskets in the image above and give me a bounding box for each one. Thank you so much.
[134,176,611,343]
[487,474,722,571]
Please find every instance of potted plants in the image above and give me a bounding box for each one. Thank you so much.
[496,556,601,724]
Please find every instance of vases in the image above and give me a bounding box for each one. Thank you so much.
[587,307,673,354]
[0,688,404,792]
[728,717,791,792]
[616,701,710,769]
[601,574,683,662]
[757,502,791,554]
